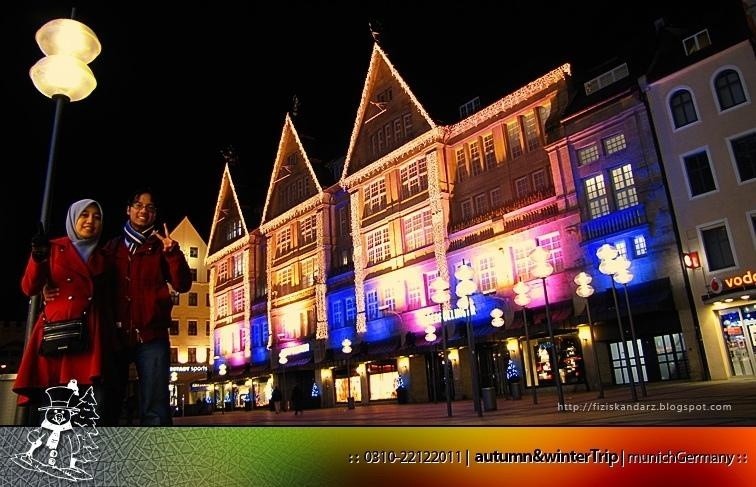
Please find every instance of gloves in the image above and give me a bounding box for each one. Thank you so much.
[31,221,51,262]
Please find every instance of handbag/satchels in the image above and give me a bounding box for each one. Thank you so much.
[43,319,91,356]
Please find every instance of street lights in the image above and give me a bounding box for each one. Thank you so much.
[455,258,483,411]
[576,268,606,400]
[342,337,355,410]
[218,364,229,415]
[596,237,648,401]
[490,307,510,398]
[278,350,290,415]
[513,276,537,405]
[425,326,440,403]
[528,237,566,410]
[168,370,181,414]
[13,7,102,429]
[432,269,455,418]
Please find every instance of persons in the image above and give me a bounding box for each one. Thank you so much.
[272,384,281,414]
[43,185,193,424]
[291,384,305,415]
[10,199,113,422]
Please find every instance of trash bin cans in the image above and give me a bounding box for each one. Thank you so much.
[511,383,521,401]
[245,401,251,411]
[348,397,355,409]
[396,388,407,405]
[225,402,231,411]
[481,387,497,412]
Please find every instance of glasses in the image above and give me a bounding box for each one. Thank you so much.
[132,202,159,212]
